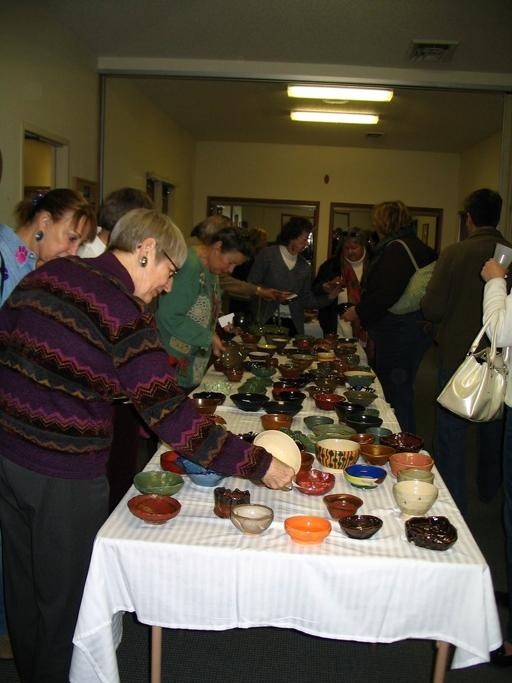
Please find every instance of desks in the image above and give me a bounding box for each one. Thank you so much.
[97,326,490,682]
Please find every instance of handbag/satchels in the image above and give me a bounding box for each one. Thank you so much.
[386,260,439,315]
[436,348,509,423]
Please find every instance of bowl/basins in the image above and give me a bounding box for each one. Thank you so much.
[283,516,331,545]
[179,294,438,520]
[134,472,184,496]
[230,504,274,535]
[337,514,383,538]
[159,450,185,475]
[127,494,181,525]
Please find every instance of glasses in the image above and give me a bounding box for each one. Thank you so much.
[162,249,180,278]
[342,231,358,238]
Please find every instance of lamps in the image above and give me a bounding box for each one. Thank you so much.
[286,81,394,125]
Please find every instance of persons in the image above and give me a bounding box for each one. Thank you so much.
[2,150,511,681]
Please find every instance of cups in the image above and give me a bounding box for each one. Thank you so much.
[493,243,512,271]
